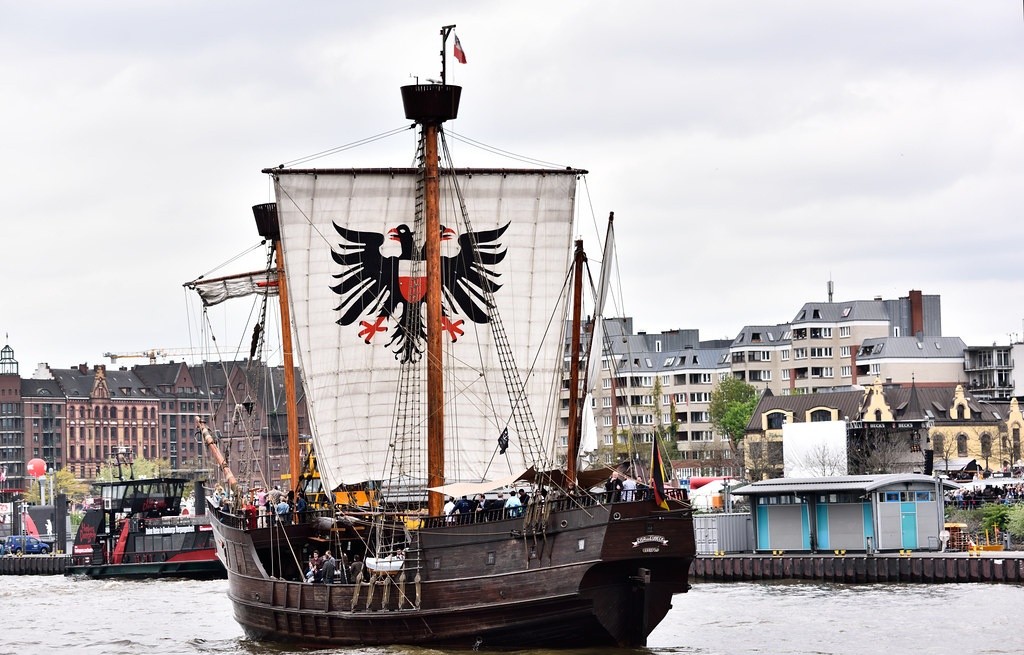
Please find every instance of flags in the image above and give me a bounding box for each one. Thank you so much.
[454,37,466,64]
[651,431,669,512]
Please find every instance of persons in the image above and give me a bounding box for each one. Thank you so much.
[444,486,560,526]
[606,472,624,503]
[943,472,1024,510]
[622,474,637,502]
[213,484,307,527]
[307,550,363,583]
[1,540,12,555]
[146,501,163,518]
[634,475,645,500]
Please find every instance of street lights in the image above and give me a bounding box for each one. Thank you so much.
[979,400,1014,479]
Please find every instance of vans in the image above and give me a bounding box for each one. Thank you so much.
[4,535,51,554]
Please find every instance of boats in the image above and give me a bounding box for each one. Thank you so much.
[65,445,231,581]
[181,22,699,648]
